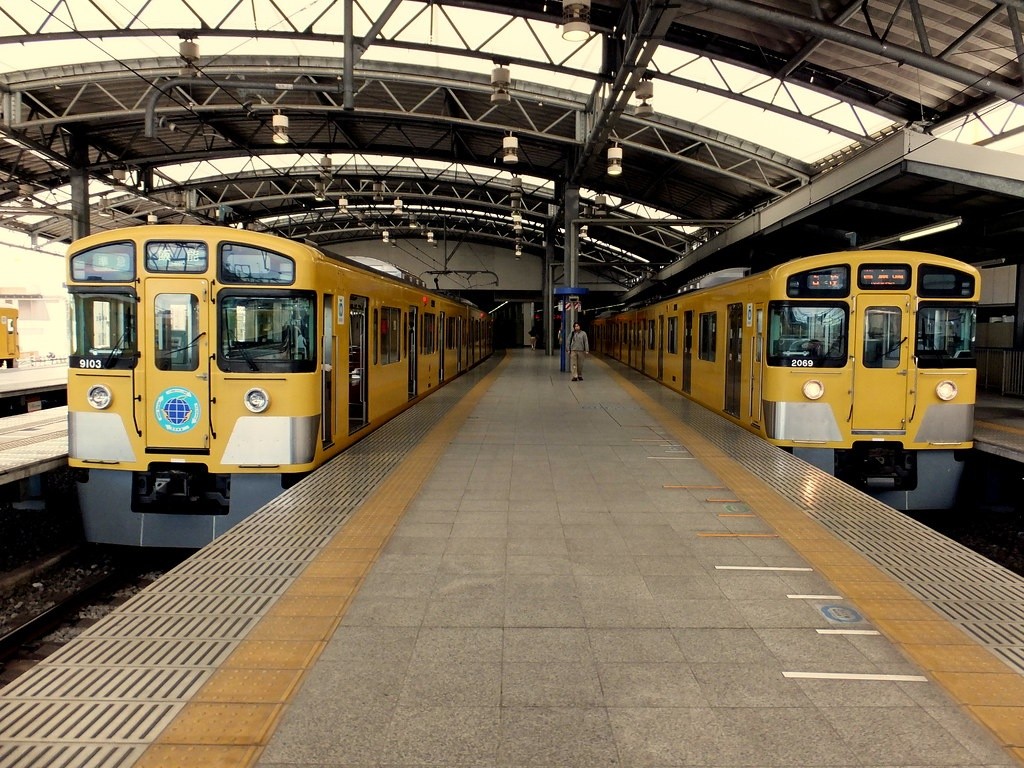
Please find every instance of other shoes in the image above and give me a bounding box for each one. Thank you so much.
[578,377,583,380]
[571,378,578,381]
[531,347,533,350]
[533,349,535,350]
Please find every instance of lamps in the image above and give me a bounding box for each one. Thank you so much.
[19,0,653,261]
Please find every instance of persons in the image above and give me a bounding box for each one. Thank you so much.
[565,322,589,381]
[528,326,538,350]
[557,322,563,339]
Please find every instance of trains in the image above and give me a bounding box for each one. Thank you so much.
[64,221,497,557]
[584,246,984,514]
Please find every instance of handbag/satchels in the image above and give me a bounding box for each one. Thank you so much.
[535,334,539,340]
